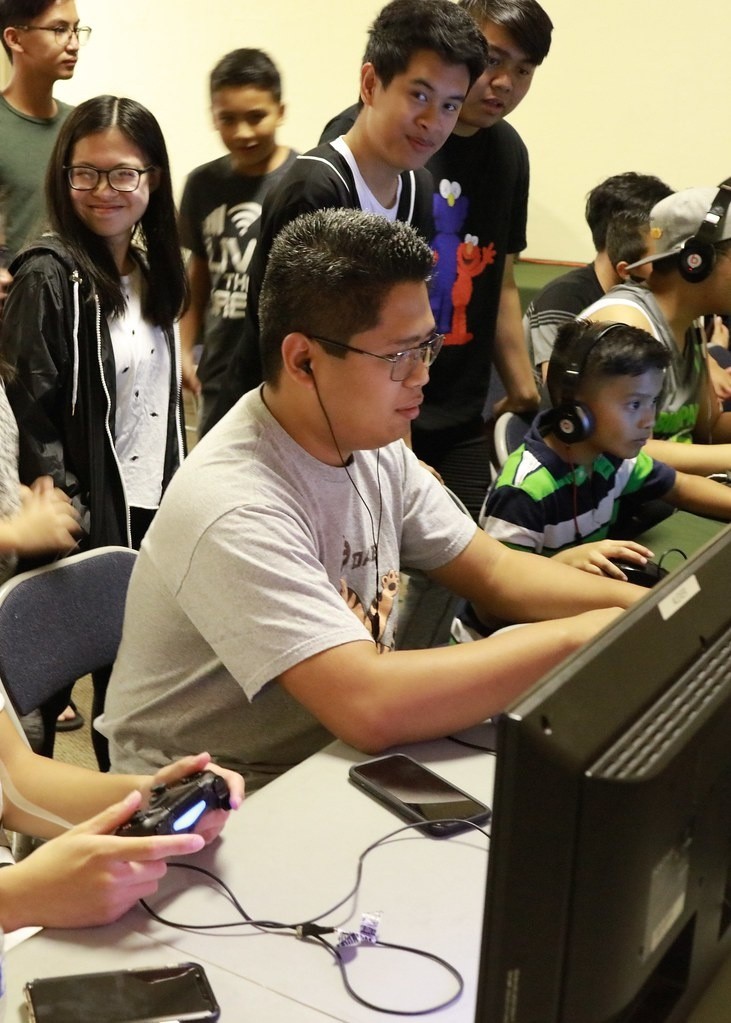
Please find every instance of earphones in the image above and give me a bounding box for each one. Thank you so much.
[302,358,313,374]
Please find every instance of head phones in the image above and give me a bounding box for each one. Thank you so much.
[550,321,630,444]
[676,177,731,283]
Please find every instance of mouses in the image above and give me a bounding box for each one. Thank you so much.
[604,558,670,587]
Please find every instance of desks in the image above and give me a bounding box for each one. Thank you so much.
[0,506,731,1023]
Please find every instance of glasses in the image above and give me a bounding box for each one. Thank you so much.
[278,330,445,381]
[4,25,91,46]
[58,165,155,192]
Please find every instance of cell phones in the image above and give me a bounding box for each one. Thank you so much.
[24,962,220,1023]
[348,752,494,837]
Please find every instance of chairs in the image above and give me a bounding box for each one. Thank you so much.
[0,545,137,772]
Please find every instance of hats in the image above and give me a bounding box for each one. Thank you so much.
[625,186,731,271]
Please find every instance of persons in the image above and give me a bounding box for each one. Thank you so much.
[176,48,299,442]
[262,0,554,649]
[1,96,193,772]
[97,208,660,797]
[1,682,247,952]
[0,214,81,586]
[1,0,92,732]
[451,168,731,646]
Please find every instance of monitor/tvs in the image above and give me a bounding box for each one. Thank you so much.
[472,521,731,1023]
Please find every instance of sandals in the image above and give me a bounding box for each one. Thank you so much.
[55,699,83,732]
